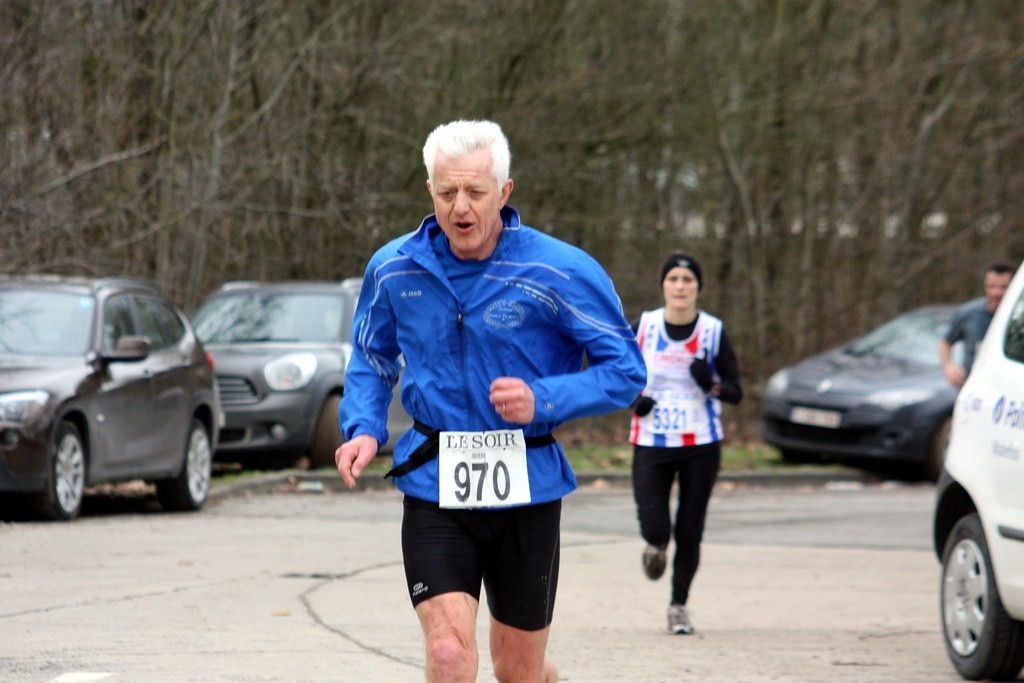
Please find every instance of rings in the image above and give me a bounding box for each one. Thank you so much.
[503,403,506,414]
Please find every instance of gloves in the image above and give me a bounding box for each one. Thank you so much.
[689,358,716,393]
[633,395,657,417]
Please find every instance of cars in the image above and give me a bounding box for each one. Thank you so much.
[763,296,985,484]
[0,274,223,520]
[929,260,1024,682]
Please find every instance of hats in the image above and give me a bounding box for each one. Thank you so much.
[660,253,703,284]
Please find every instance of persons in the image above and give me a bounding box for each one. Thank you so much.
[629,252,743,634]
[334,119,648,683]
[939,264,1017,391]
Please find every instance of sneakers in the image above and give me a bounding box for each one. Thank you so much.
[667,603,694,635]
[642,544,667,580]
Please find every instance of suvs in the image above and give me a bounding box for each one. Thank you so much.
[189,279,413,470]
